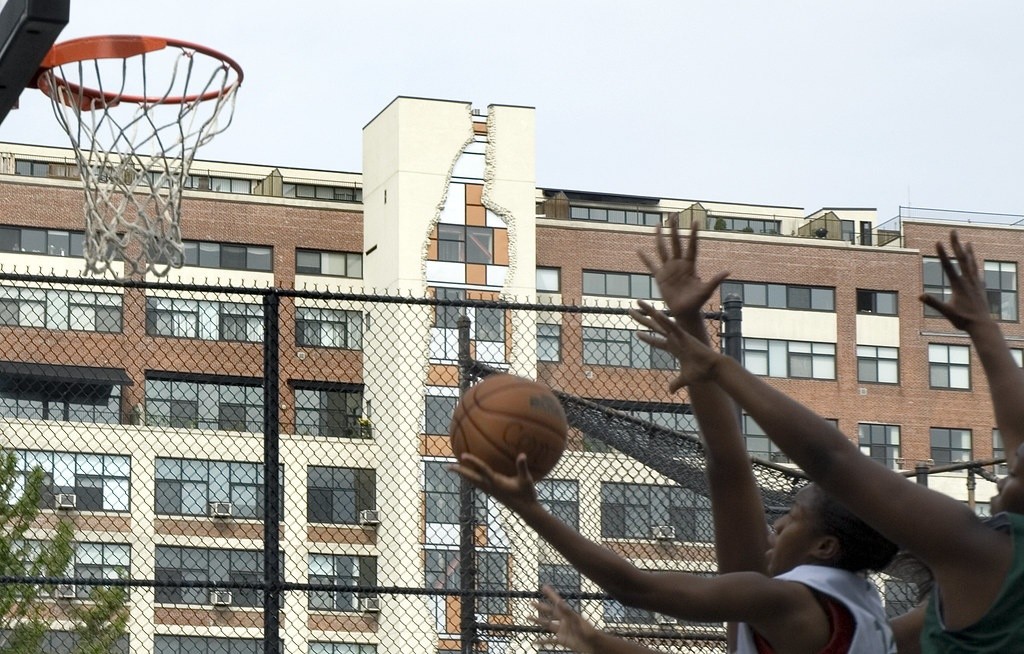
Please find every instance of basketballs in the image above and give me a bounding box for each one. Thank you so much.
[449,373,573,485]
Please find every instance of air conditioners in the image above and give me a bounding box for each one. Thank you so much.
[58,584,76,598]
[359,510,382,525]
[655,612,677,624]
[211,502,232,515]
[654,526,675,540]
[56,494,75,507]
[213,591,232,604]
[361,598,381,611]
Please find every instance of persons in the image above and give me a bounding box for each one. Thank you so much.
[449,215,1023,654]
